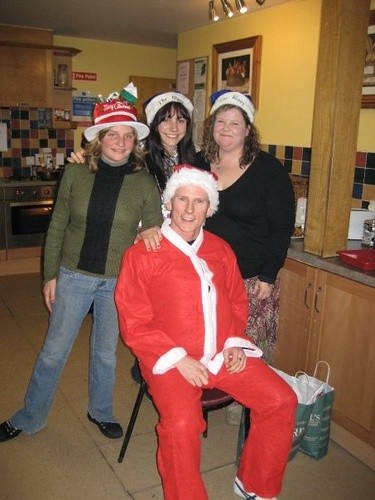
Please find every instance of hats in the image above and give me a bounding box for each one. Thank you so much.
[210,89,256,123]
[161,163,220,225]
[142,91,194,127]
[84,81,150,142]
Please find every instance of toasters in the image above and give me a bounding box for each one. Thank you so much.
[348,207,374,239]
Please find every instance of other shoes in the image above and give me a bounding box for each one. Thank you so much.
[233,477,279,500]
[226,402,243,425]
[88,413,123,439]
[0,420,22,443]
[132,358,142,383]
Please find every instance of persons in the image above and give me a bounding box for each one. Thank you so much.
[66,89,204,405]
[114,164,298,500]
[189,89,297,427]
[1,82,166,447]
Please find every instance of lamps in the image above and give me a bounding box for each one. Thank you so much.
[209,0,265,21]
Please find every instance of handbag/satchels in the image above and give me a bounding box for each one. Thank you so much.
[235,365,328,467]
[295,360,335,460]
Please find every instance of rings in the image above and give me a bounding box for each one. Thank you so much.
[239,358,242,361]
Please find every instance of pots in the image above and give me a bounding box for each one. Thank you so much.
[38,172,60,181]
[12,167,30,177]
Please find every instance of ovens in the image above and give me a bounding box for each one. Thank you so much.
[3,185,56,250]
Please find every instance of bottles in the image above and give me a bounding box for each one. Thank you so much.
[361,219,375,250]
[57,66,69,88]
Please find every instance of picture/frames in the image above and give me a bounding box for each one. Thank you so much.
[212,35,262,111]
[360,10,375,108]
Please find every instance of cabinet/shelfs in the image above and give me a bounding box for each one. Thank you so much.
[271,257,375,472]
[0,24,83,129]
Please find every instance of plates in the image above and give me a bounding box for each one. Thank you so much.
[336,248,375,271]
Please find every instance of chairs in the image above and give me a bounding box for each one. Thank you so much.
[118,357,250,463]
